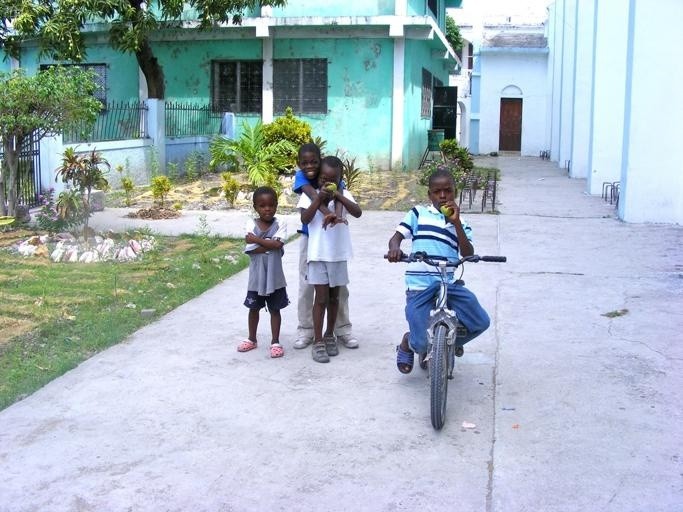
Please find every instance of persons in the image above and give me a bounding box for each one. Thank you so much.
[291,143,362,363]
[235,186,290,357]
[387,169,491,374]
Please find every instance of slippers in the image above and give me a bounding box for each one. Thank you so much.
[455,346,464,357]
[397,333,414,374]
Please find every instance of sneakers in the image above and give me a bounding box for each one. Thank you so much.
[337,334,359,348]
[294,337,313,349]
[312,337,338,363]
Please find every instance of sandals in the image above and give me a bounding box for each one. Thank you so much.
[237,339,257,352]
[270,343,284,358]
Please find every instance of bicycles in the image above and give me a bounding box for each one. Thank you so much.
[384,252,507,431]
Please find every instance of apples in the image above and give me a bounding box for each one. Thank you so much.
[326,183,338,192]
[440,205,452,217]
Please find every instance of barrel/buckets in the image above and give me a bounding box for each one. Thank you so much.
[427,129,445,151]
